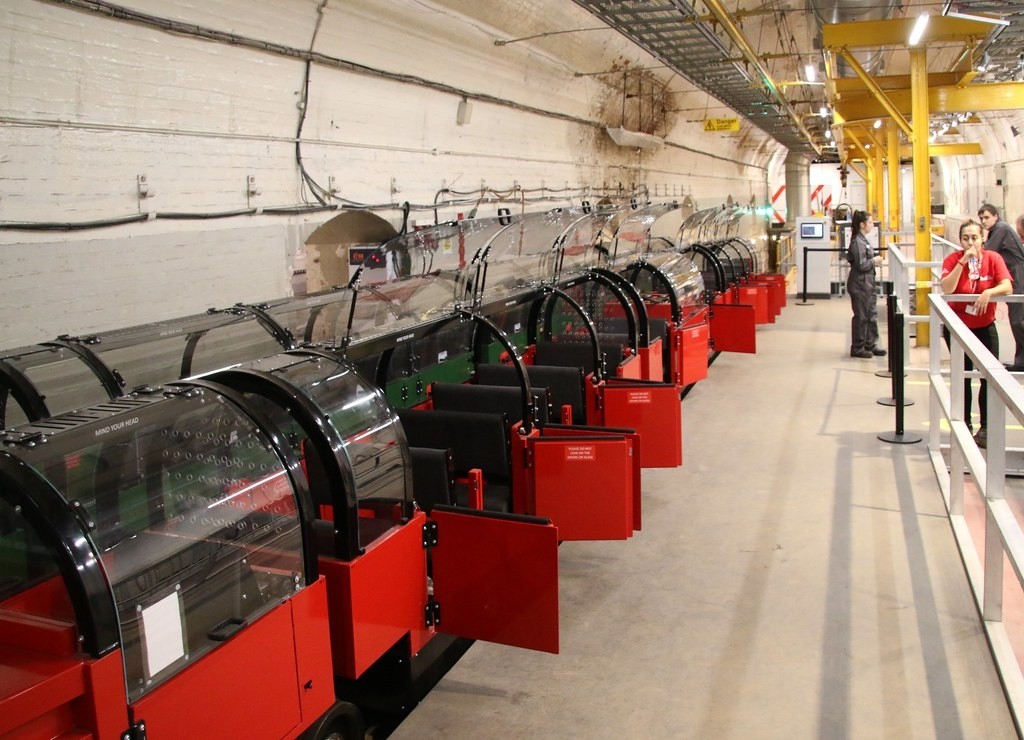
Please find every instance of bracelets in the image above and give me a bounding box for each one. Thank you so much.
[957,260,965,266]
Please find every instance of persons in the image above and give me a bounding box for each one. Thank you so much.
[846,209,890,356]
[979,204,1024,371]
[941,218,1013,449]
[1015,215,1024,243]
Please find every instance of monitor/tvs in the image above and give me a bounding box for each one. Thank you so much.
[801,223,823,238]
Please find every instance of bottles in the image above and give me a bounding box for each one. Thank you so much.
[968,255,979,279]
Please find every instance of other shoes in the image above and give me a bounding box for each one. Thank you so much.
[964,420,973,434]
[1003,363,1024,371]
[973,427,987,451]
[851,345,887,358]
